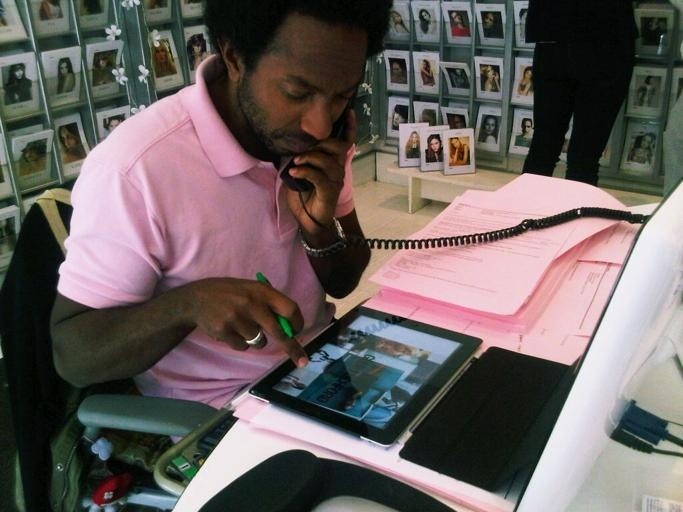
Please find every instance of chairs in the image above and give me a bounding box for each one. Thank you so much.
[15,178,238,511]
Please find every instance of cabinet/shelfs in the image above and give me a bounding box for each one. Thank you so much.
[372,0,683,184]
[0,1,216,242]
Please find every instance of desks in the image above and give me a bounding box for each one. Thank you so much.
[156,203,683,512]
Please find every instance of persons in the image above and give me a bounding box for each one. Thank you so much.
[1,105,130,199]
[1,0,203,43]
[3,25,212,120]
[309,350,336,365]
[378,0,533,167]
[0,197,37,269]
[337,364,384,411]
[50,0,394,472]
[524,1,641,185]
[280,375,305,389]
[339,327,429,361]
[596,18,681,165]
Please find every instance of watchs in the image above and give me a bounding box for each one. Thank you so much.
[296,216,350,258]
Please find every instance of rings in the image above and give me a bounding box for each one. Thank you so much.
[244,328,266,347]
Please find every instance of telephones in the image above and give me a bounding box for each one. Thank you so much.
[281,59,366,191]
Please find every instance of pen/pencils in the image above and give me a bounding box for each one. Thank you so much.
[256,272,293,340]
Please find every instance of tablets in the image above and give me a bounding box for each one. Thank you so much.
[246,305,484,449]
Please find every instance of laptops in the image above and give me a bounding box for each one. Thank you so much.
[399,176,683,512]
